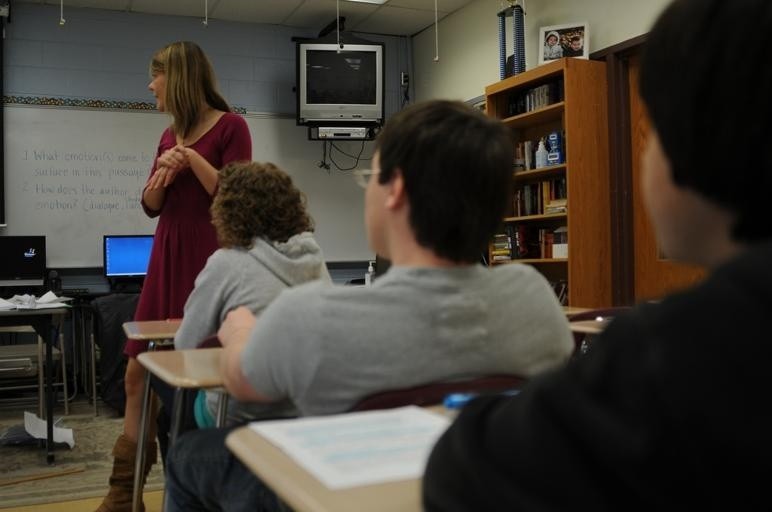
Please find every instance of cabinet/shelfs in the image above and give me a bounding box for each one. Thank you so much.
[486,57,610,307]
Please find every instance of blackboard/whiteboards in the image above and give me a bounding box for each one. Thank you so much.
[0,95,378,276]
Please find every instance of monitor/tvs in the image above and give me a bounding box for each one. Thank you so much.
[299,43,383,124]
[0,236,46,298]
[103,235,155,293]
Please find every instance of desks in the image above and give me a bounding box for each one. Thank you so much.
[0,269,641,512]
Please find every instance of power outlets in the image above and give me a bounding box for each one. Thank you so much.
[400,71,409,87]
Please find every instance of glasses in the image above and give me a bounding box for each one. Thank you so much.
[353,168,385,189]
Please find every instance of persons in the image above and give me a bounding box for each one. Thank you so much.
[420,1,771,510]
[161,98,578,512]
[155,161,333,476]
[543,30,583,60]
[94,41,253,512]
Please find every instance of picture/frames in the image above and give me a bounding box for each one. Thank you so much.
[538,22,590,65]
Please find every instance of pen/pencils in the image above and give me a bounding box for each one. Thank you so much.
[443,389,522,409]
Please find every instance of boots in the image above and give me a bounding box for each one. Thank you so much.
[95,434,158,512]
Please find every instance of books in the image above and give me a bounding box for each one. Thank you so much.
[487,178,568,262]
[523,84,559,112]
[553,278,569,308]
